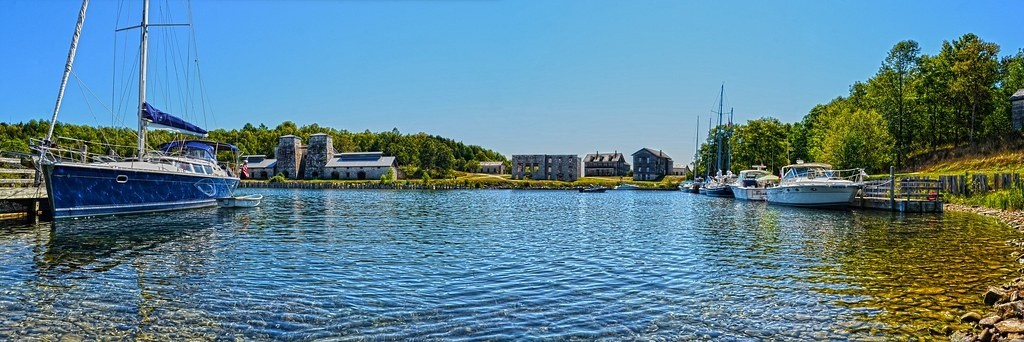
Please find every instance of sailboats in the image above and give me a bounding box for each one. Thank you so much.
[27,0,242,219]
[679,81,739,198]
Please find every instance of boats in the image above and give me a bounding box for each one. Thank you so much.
[614,184,639,191]
[214,193,263,209]
[767,161,863,206]
[580,184,607,193]
[729,171,777,201]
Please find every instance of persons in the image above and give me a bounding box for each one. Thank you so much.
[241,159,249,180]
